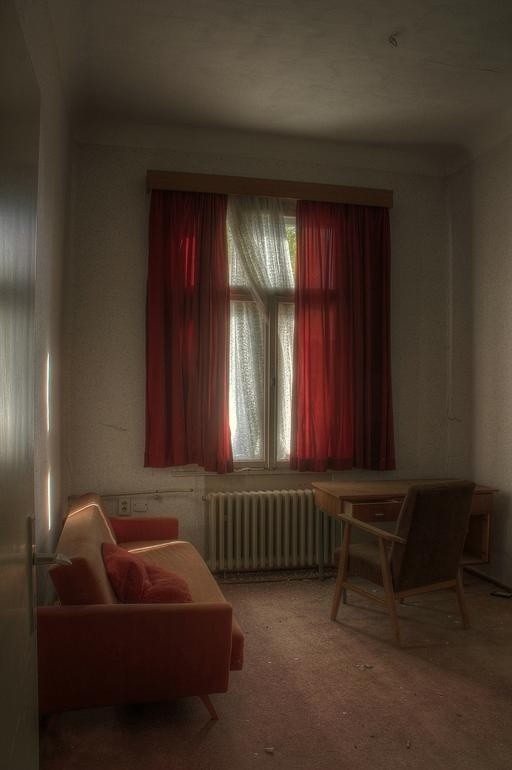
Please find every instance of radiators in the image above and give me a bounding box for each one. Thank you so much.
[200,489,340,573]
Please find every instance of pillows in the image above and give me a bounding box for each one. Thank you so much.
[99,541,193,606]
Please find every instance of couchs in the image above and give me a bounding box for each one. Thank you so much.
[33,491,243,721]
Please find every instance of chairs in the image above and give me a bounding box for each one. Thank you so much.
[329,479,477,644]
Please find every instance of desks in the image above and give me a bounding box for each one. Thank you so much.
[310,475,501,582]
